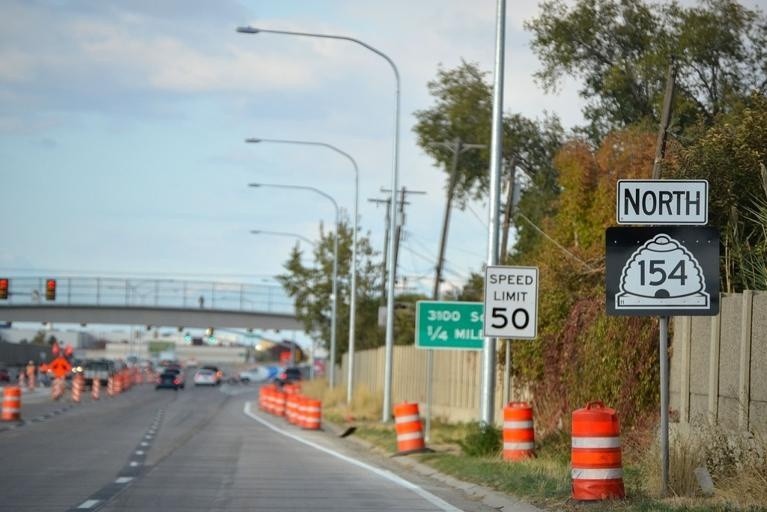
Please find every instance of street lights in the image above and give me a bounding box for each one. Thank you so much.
[235,23,403,428]
[248,228,318,248]
[241,133,361,403]
[247,179,339,391]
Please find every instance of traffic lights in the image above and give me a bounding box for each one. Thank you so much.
[0,278,8,299]
[45,278,57,301]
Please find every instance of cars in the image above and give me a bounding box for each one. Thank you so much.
[40,357,313,392]
[1,367,12,383]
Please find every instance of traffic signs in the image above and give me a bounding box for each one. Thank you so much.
[484,264,539,338]
[416,301,485,350]
